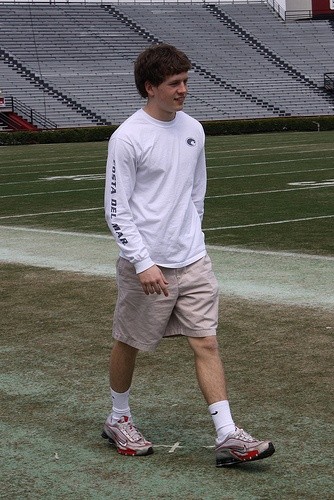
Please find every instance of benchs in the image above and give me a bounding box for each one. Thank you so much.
[0,0,334,130]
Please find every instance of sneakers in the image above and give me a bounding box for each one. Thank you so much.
[101,414,154,456]
[215,427,275,467]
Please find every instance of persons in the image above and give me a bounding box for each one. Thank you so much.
[100,43,276,467]
